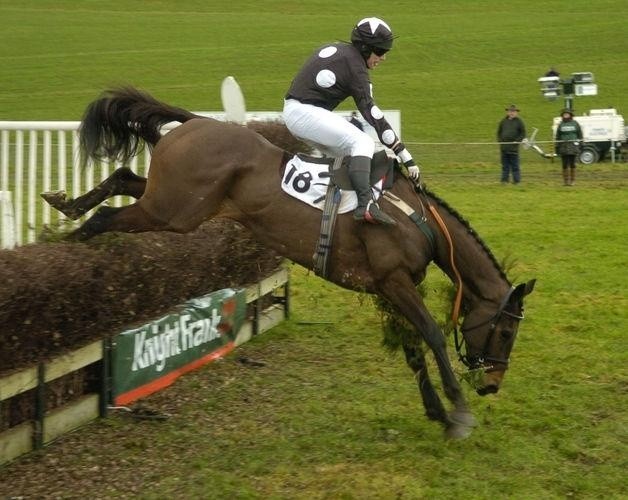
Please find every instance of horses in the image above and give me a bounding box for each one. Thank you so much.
[36,82,537,449]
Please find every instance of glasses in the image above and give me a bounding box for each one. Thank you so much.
[370,47,387,56]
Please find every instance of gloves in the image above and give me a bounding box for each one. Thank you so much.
[408,165,420,181]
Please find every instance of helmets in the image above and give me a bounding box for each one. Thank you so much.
[351,17,393,49]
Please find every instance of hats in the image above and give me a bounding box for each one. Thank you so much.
[505,104,520,112]
[561,110,574,117]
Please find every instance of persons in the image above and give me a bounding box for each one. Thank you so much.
[497,104,526,185]
[283,16,420,228]
[554,107,583,187]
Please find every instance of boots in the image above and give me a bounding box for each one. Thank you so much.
[563,168,576,186]
[349,156,397,226]
